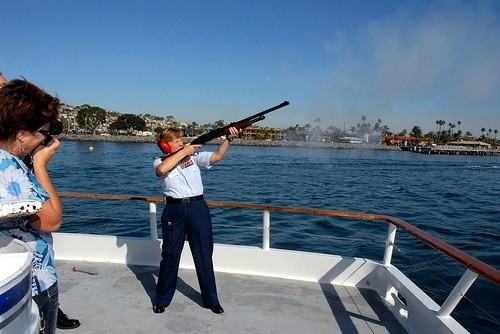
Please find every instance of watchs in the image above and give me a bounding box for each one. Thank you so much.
[225,135,233,142]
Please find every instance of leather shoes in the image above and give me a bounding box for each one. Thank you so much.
[154,305,165,313]
[56,307,81,329]
[210,304,224,314]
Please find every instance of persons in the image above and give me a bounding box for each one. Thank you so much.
[0,78,62,334]
[153,126,243,313]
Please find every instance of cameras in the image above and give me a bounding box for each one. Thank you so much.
[41,120,64,146]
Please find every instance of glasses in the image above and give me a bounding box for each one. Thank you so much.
[34,128,53,142]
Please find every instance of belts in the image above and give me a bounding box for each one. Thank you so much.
[166,195,204,203]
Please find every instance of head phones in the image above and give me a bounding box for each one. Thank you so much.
[158,141,171,155]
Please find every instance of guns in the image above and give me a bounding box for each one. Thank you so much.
[189,100,289,145]
